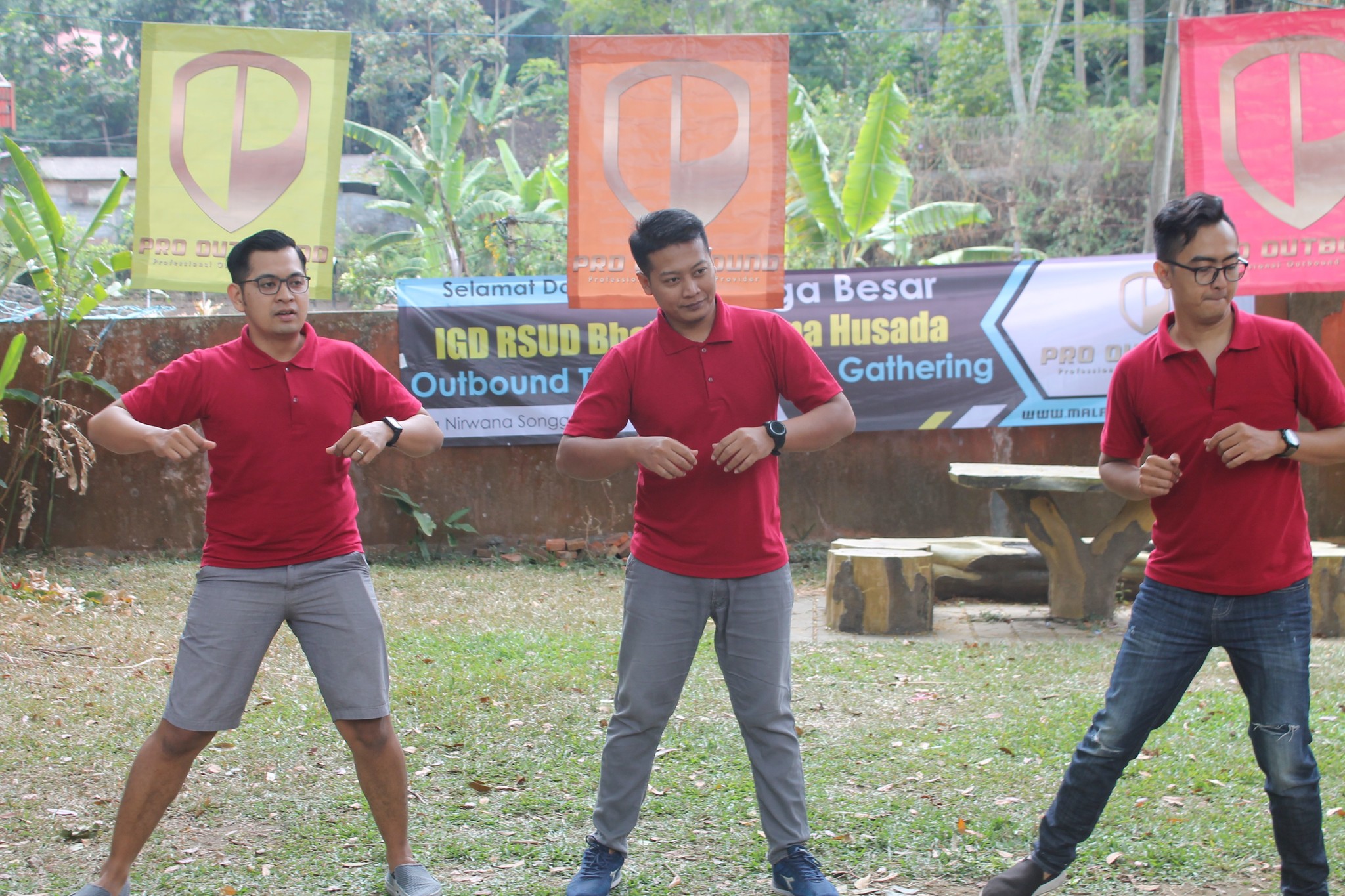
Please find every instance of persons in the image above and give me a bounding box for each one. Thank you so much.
[61,231,446,895]
[553,210,856,895]
[981,192,1345,896]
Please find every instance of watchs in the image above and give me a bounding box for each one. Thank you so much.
[762,420,786,456]
[380,417,404,448]
[1276,428,1301,459]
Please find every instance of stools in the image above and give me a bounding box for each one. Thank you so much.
[828,537,932,559]
[1307,542,1345,639]
[824,546,937,636]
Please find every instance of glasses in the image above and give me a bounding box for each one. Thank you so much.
[1164,257,1248,286]
[237,275,310,295]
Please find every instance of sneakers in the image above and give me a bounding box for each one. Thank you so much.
[979,853,1065,896]
[383,863,444,896]
[770,844,840,896]
[567,835,624,896]
[67,876,131,896]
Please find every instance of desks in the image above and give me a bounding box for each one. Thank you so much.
[948,458,1157,627]
